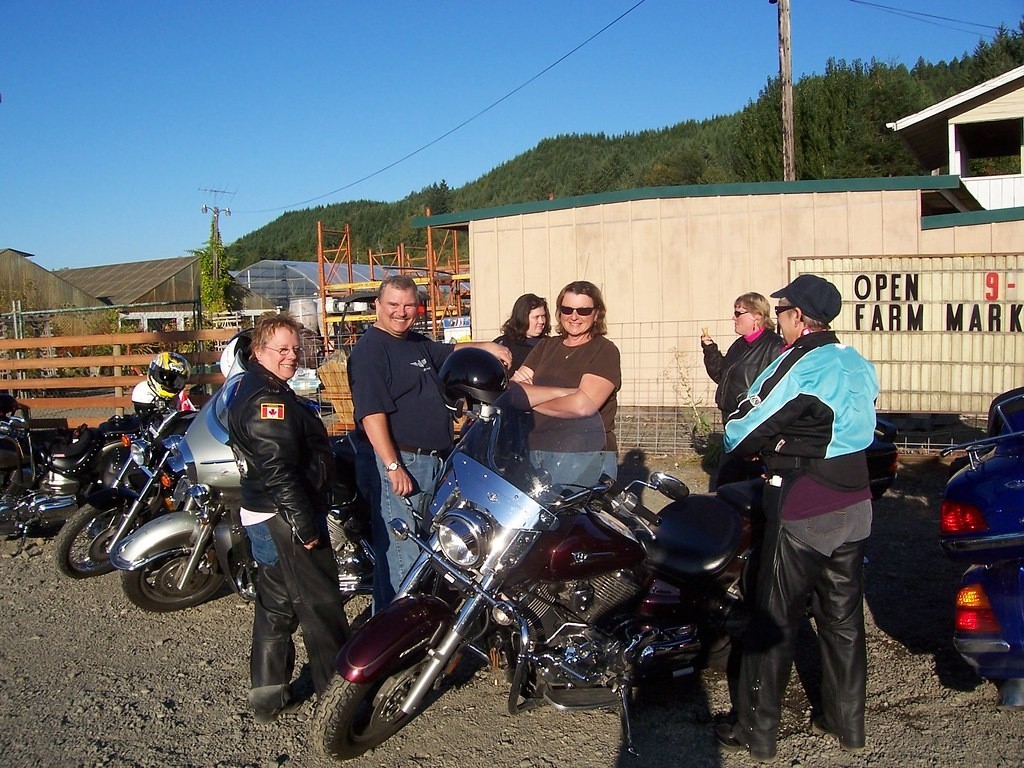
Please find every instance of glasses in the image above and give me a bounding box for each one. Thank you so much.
[265,347,299,355]
[775,306,804,322]
[561,306,594,316]
[735,311,748,318]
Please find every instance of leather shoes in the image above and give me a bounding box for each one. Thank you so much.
[714,723,775,762]
[812,716,865,751]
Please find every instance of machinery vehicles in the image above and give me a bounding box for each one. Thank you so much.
[316,291,431,412]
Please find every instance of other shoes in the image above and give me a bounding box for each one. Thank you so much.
[255,695,301,720]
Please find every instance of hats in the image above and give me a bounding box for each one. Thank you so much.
[770,275,842,323]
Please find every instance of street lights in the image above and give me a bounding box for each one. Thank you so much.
[202,204,231,280]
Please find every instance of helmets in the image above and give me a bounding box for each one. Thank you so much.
[220,328,254,378]
[147,352,190,400]
[438,348,507,412]
[132,381,159,406]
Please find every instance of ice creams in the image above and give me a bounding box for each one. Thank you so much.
[701,327,708,336]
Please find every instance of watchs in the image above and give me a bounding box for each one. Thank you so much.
[384,461,401,472]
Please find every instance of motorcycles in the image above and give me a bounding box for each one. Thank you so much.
[941,387,1024,709]
[310,373,900,762]
[108,328,377,612]
[0,394,165,557]
[52,382,200,579]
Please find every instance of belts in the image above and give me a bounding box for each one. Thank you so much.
[394,444,438,456]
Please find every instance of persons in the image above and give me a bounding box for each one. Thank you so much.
[701,292,786,492]
[509,281,622,494]
[493,294,552,379]
[715,275,878,762]
[228,312,352,725]
[347,275,512,617]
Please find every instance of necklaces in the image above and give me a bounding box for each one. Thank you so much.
[560,337,586,360]
